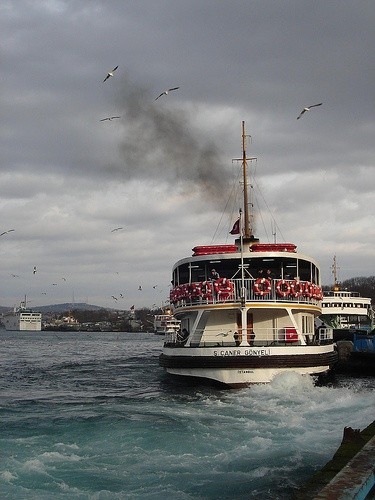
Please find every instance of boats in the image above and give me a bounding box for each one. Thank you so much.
[4,293,41,331]
[153,309,181,334]
[160,120,338,389]
[323,254,375,373]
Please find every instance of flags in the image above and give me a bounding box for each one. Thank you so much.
[228,219,240,235]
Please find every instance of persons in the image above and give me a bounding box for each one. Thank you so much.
[267,268,277,280]
[253,267,265,279]
[170,279,178,287]
[181,328,189,343]
[208,268,221,280]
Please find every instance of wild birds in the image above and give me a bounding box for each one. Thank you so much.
[11,274,18,277]
[153,285,158,289]
[129,305,134,310]
[53,284,56,286]
[103,66,118,82]
[100,116,120,121]
[0,229,14,237]
[33,267,37,275]
[42,293,46,294]
[111,296,116,300]
[138,286,142,291]
[216,330,232,336]
[112,227,122,232]
[120,294,123,298]
[155,87,179,101]
[62,278,66,281]
[138,299,170,331]
[297,103,322,121]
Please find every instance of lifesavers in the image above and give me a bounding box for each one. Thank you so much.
[169,283,197,303]
[214,277,232,295]
[197,281,212,297]
[304,281,324,301]
[276,280,290,297]
[291,281,304,297]
[253,278,271,297]
